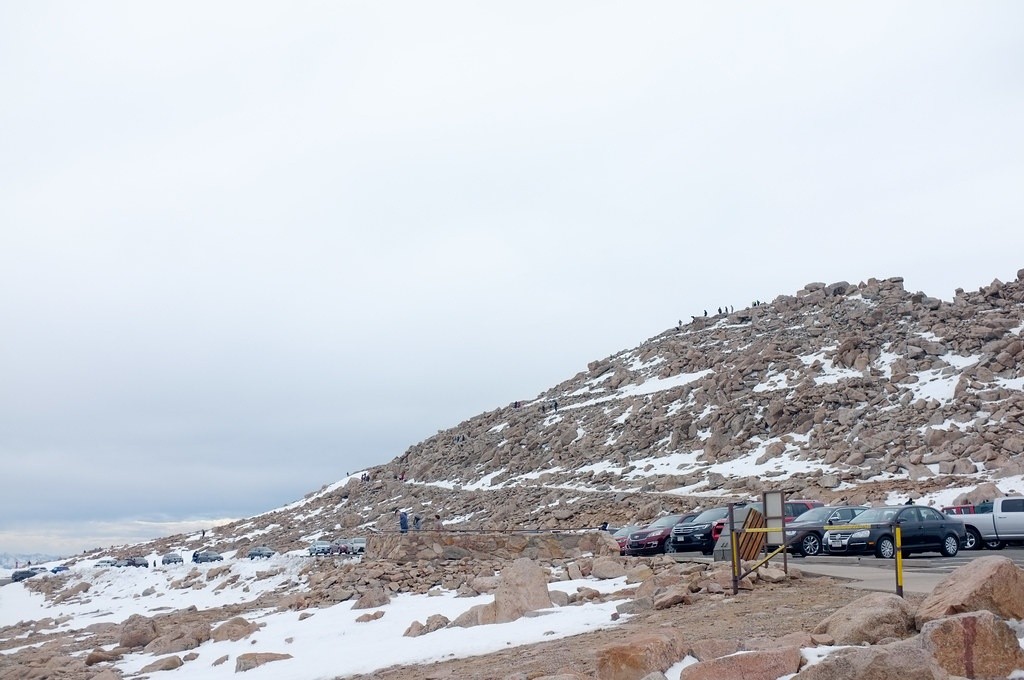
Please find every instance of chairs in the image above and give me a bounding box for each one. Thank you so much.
[887,513,893,520]
[905,511,915,523]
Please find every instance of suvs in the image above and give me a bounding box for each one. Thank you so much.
[611,524,651,555]
[710,499,825,555]
[667,504,743,553]
[624,513,702,556]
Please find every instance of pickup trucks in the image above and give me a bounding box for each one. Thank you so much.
[944,495,1024,550]
[940,501,1007,550]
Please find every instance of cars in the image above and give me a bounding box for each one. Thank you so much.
[161,552,184,564]
[51,566,69,574]
[131,554,149,568]
[308,540,332,557]
[193,549,223,563]
[93,560,116,567]
[247,546,276,560]
[329,538,352,555]
[784,505,871,556]
[820,503,968,558]
[350,537,367,554]
[12,570,36,582]
[28,567,48,575]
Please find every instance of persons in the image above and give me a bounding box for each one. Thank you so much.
[202,529,205,540]
[754,300,760,307]
[27,561,31,568]
[731,305,733,313]
[679,319,682,326]
[413,515,422,528]
[433,515,444,529]
[538,401,558,413]
[395,509,408,533]
[704,310,707,316]
[725,306,728,314]
[84,550,86,555]
[347,472,349,476]
[191,551,197,562]
[514,401,520,408]
[361,473,370,482]
[718,307,722,314]
[675,327,679,331]
